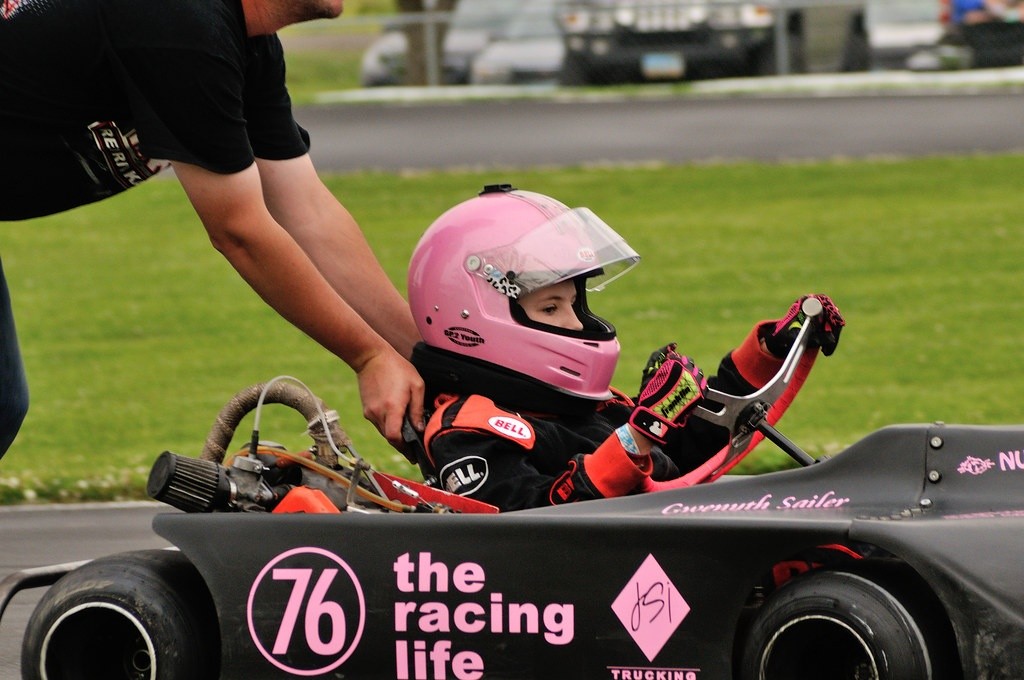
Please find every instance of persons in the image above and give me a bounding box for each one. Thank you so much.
[0,1,424,459]
[407,183,845,513]
[952,0,1023,68]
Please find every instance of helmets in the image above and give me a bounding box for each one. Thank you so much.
[407,185,620,401]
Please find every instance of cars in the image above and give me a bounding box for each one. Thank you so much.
[356,0,977,86]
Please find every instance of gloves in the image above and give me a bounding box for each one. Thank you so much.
[628,343,709,445]
[765,290,846,359]
